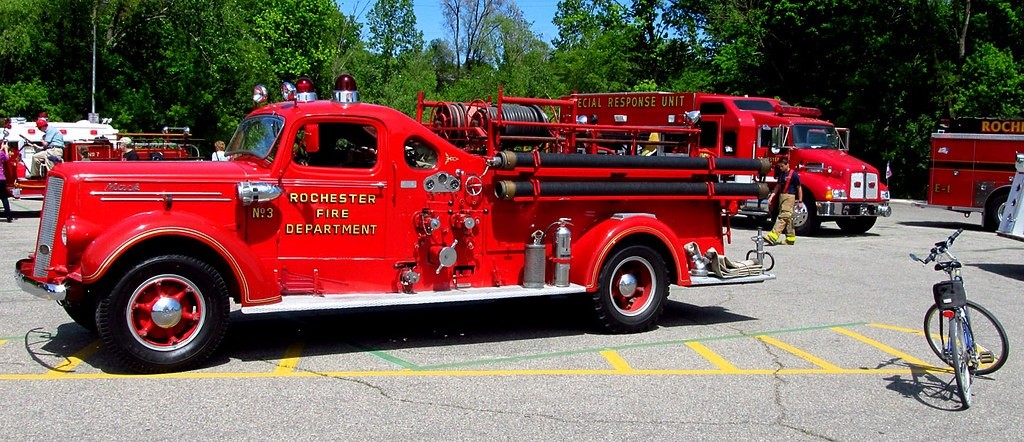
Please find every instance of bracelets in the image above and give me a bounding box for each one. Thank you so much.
[799,201,802,203]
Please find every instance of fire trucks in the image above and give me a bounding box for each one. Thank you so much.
[556,85,893,239]
[0,107,207,217]
[8,73,785,375]
[925,112,1024,243]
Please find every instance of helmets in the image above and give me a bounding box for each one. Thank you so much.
[36,120,47,129]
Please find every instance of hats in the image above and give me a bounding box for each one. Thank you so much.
[116,137,131,144]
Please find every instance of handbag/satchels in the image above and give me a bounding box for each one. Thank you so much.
[933,280,967,311]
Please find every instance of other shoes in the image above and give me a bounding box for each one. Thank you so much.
[7,219,12,222]
[25,175,42,180]
[763,237,775,245]
[780,239,794,245]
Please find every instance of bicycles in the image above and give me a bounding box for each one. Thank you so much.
[921,226,1012,412]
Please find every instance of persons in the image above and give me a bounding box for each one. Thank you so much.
[0,140,13,223]
[211,140,231,162]
[763,157,803,246]
[24,119,65,180]
[77,145,92,161]
[119,137,139,161]
